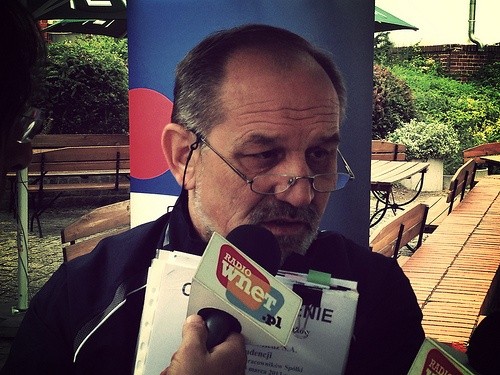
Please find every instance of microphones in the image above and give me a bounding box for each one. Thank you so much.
[186,222,302,350]
[406,313,500,375]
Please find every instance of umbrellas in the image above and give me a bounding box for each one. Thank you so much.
[374,5,419,33]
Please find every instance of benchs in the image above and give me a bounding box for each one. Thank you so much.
[369,203,430,260]
[422,159,477,233]
[463,142,500,182]
[372,140,407,191]
[6,134,130,262]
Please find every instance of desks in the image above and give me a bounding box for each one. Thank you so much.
[480,155,500,175]
[369,160,431,228]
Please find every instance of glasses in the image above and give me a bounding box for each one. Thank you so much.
[177,120,356,195]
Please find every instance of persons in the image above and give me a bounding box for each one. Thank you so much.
[0,23,423,375]
